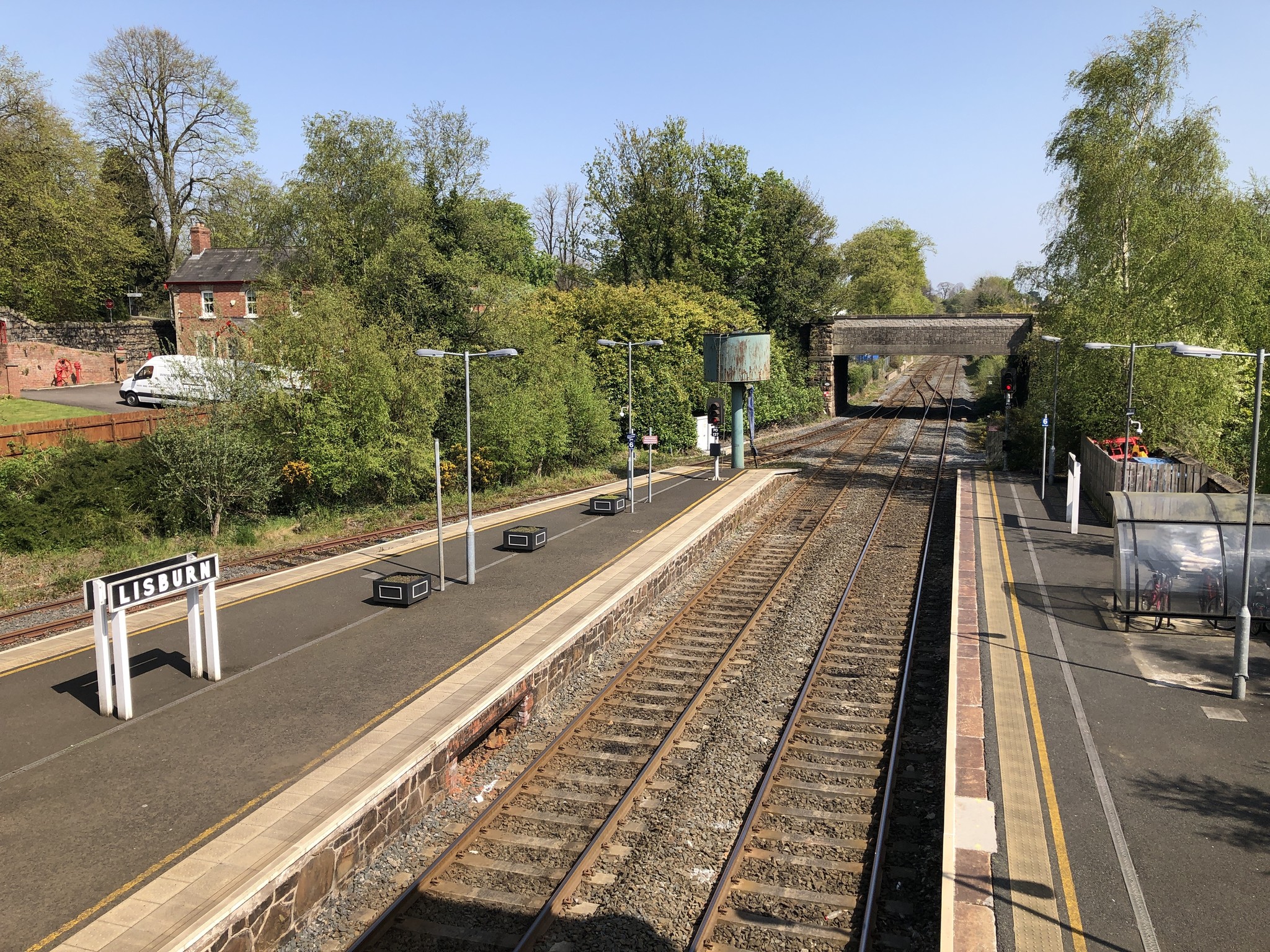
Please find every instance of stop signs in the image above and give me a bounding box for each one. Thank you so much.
[105,298,114,308]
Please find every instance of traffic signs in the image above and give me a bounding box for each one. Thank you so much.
[127,293,143,297]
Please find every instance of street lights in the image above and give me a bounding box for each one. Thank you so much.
[1170,345,1270,700]
[1085,340,1184,493]
[1042,335,1061,486]
[596,339,664,498]
[413,348,518,585]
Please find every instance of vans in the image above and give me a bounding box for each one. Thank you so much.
[121,355,313,408]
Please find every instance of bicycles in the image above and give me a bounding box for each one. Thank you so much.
[1249,565,1269,634]
[1199,567,1242,631]
[1142,569,1188,629]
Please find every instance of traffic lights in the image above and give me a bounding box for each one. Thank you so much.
[1001,368,1016,392]
[707,398,724,424]
